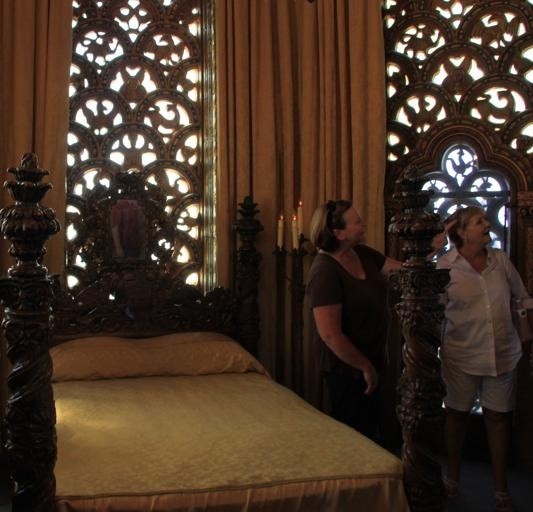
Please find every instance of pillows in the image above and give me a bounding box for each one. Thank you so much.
[46,331,270,383]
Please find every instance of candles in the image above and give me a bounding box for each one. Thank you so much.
[276,200,306,250]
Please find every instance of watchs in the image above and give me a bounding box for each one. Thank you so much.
[516,308,528,318]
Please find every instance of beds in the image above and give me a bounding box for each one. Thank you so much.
[0,153,448,512]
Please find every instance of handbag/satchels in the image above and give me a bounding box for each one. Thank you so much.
[509,295,533,344]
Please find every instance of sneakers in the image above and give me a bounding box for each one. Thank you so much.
[447,478,514,512]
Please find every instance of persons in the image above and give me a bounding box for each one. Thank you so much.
[109,200,144,259]
[429,203,533,512]
[303,198,405,438]
